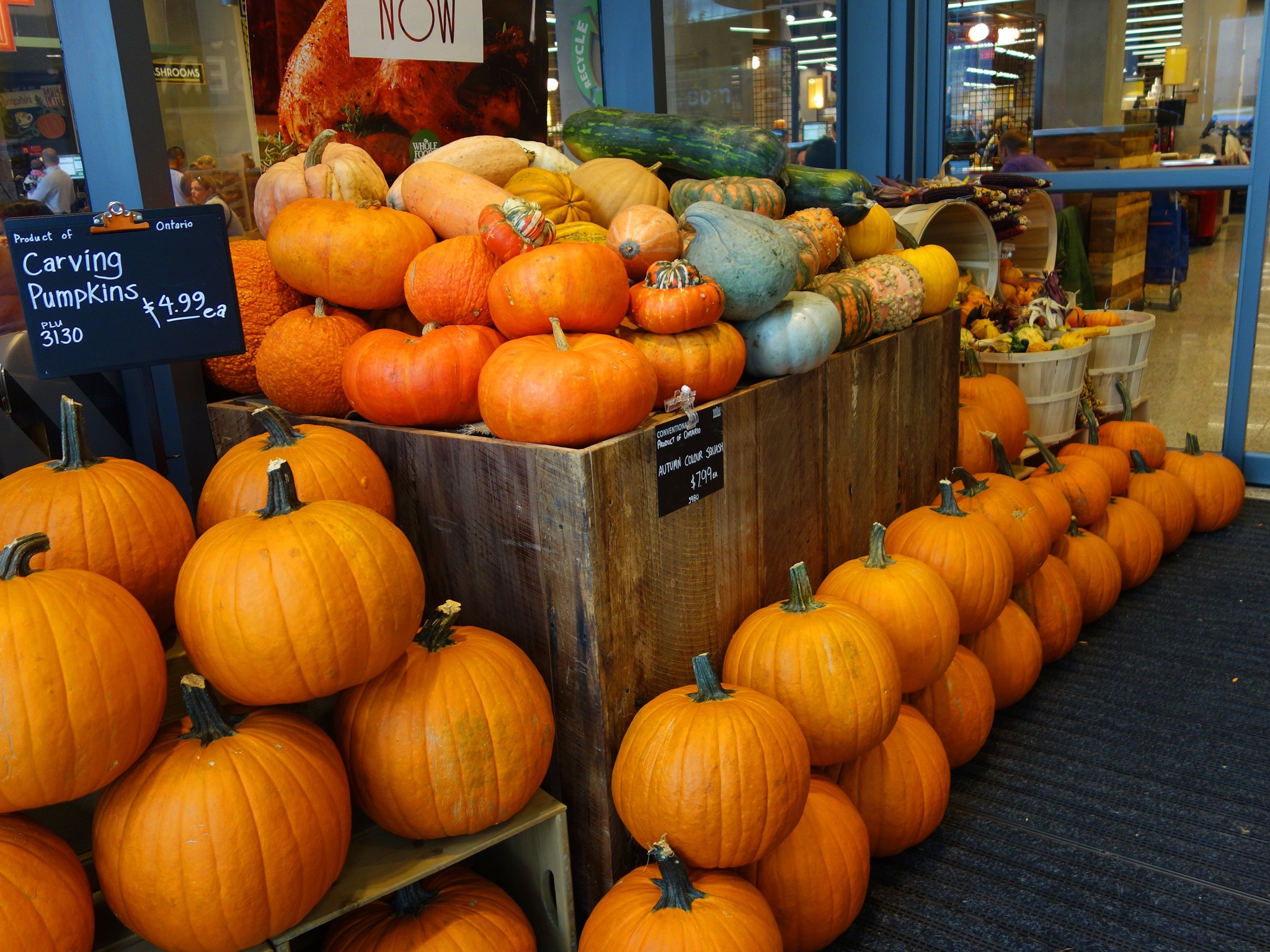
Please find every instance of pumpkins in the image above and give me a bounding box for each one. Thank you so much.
[1,103,1246,949]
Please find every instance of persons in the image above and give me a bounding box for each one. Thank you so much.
[190,174,246,238]
[166,146,194,207]
[997,130,1065,217]
[23,159,46,191]
[27,147,77,215]
[752,89,1051,182]
[188,155,217,180]
